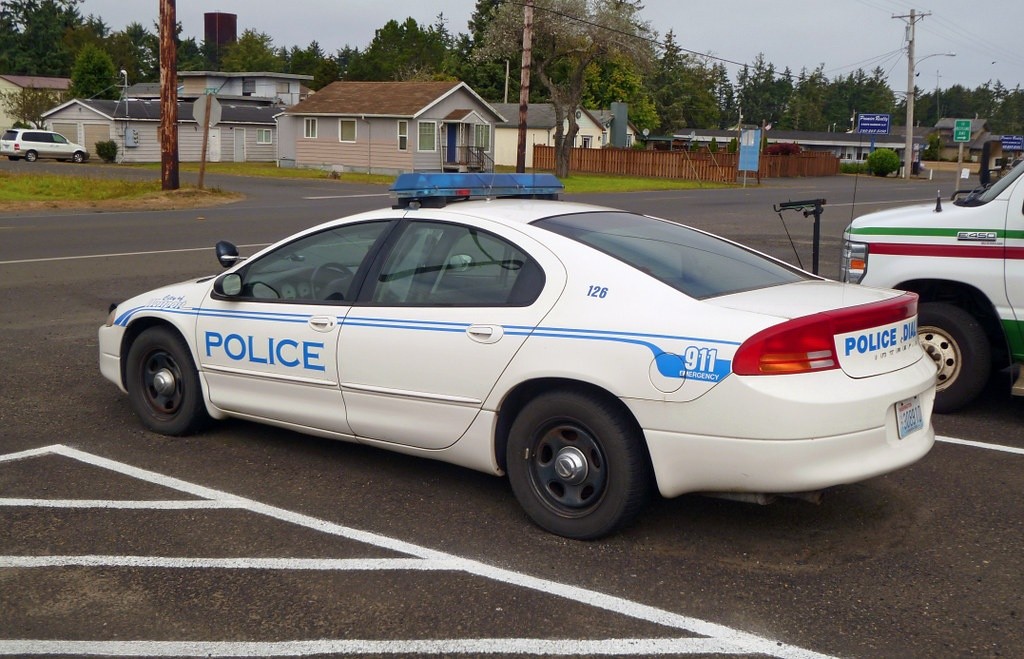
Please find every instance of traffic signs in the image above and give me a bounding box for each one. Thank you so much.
[954,120,971,142]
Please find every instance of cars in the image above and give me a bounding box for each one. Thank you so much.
[96,170,940,543]
[0,129,90,163]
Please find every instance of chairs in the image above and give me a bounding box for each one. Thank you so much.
[371,232,429,302]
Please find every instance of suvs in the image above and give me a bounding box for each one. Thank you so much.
[840,157,1024,415]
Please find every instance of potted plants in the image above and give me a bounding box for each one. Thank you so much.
[95,140,118,164]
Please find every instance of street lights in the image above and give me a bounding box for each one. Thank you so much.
[122,70,129,115]
[903,52,957,177]
